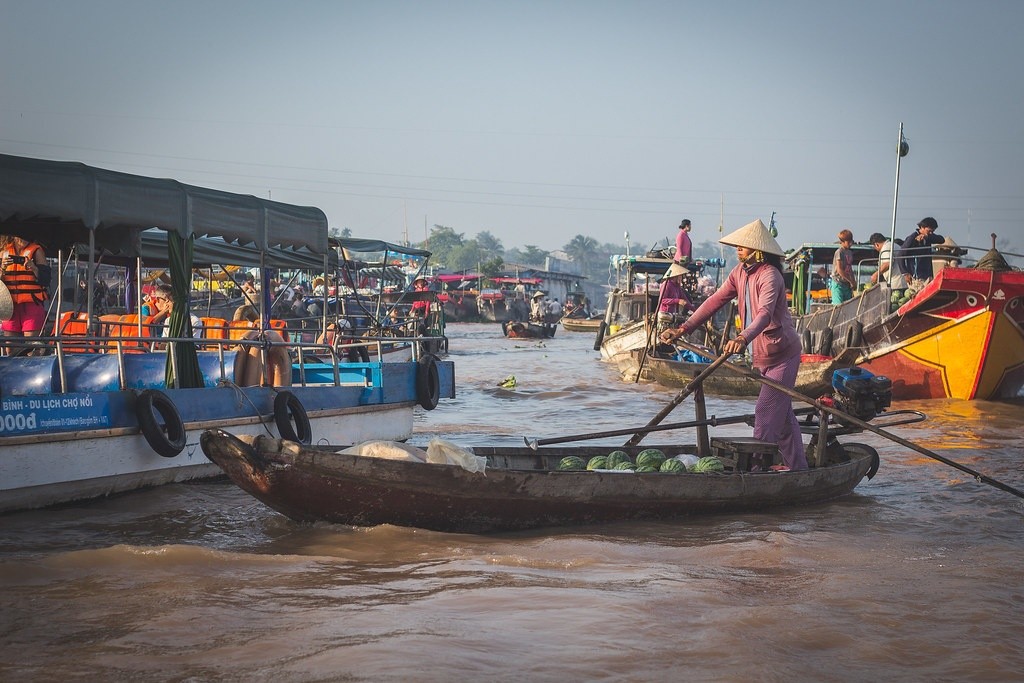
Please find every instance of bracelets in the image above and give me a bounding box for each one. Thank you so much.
[0,267,6,271]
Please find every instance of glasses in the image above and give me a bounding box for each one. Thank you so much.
[157,296,169,303]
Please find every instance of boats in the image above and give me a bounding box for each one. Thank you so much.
[200,364,926,536]
[1,152,1024,519]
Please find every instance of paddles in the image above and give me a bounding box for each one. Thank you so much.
[624,339,745,447]
[644,271,649,337]
[634,269,672,383]
[663,331,1024,500]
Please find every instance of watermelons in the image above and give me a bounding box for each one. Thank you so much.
[856,282,916,312]
[559,449,725,472]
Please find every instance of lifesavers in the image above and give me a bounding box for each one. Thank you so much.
[232,329,293,388]
[232,304,258,322]
[844,321,863,350]
[315,323,351,353]
[594,321,606,351]
[545,322,551,335]
[134,389,187,458]
[512,323,525,333]
[273,390,312,447]
[551,324,557,337]
[802,329,811,354]
[501,321,507,336]
[818,327,833,355]
[348,340,370,363]
[417,354,441,410]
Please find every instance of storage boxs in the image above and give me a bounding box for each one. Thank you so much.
[289,333,315,343]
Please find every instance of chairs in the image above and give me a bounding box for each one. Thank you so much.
[49,312,290,354]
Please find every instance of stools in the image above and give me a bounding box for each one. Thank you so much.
[710,437,779,473]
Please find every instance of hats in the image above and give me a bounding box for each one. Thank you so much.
[934,237,960,247]
[663,263,690,280]
[411,276,428,288]
[0,280,14,322]
[246,272,252,280]
[718,219,786,257]
[533,290,545,298]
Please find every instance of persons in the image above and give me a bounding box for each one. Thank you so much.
[567,304,589,318]
[384,306,404,330]
[548,298,563,323]
[147,284,173,340]
[831,230,855,305]
[660,218,809,473]
[158,292,203,349]
[534,291,546,322]
[407,272,432,330]
[242,275,257,298]
[291,294,322,317]
[657,263,696,315]
[897,217,946,294]
[0,223,52,357]
[869,232,909,297]
[515,281,526,292]
[673,219,692,264]
